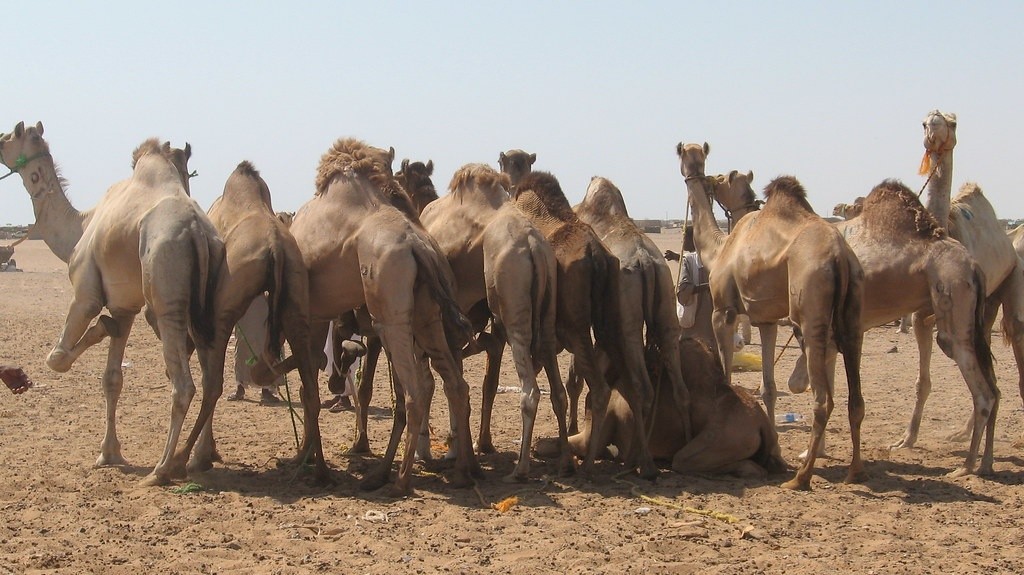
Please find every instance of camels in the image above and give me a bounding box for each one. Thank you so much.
[0,109,1024,491]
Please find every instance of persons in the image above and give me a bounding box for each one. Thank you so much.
[0,364,33,394]
[227,291,286,403]
[324,319,362,412]
[664,226,721,348]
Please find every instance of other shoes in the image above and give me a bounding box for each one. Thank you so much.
[262,393,279,403]
[329,402,354,411]
[320,400,334,408]
[228,392,245,401]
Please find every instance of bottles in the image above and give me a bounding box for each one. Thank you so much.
[774,412,804,423]
